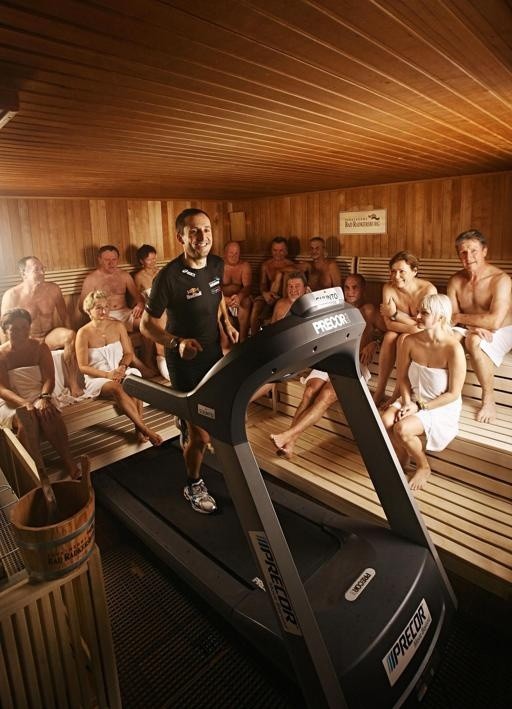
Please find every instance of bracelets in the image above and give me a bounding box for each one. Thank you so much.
[372,340,380,351]
[416,401,428,411]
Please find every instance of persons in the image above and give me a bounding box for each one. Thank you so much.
[372,251,438,416]
[1,245,168,478]
[447,230,512,424]
[217,236,342,350]
[138,208,240,513]
[269,274,387,460]
[381,293,466,491]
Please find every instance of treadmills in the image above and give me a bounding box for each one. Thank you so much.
[76,286,461,709]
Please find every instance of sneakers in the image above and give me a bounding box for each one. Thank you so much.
[174,414,190,450]
[184,476,218,514]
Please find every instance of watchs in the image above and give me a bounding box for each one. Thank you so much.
[391,312,398,321]
[168,337,180,349]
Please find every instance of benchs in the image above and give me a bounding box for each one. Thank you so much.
[309,474,512,593]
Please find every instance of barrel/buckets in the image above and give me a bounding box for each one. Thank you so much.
[2,454,98,585]
